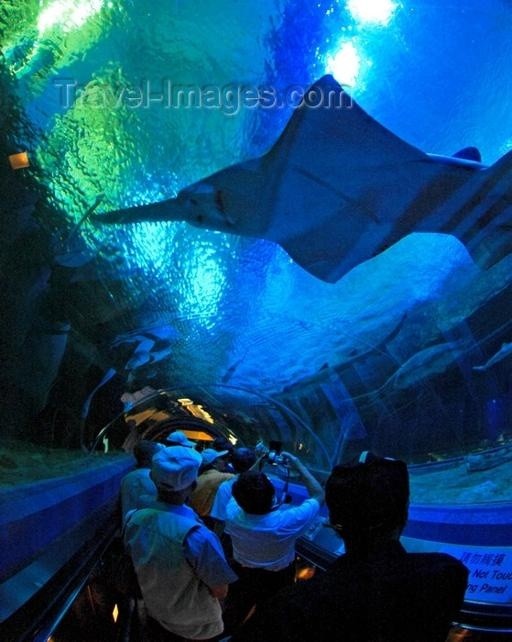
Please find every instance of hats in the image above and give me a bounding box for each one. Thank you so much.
[134,430,229,493]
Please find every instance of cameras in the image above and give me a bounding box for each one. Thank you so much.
[274,455,288,464]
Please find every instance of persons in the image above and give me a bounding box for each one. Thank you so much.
[119,429,471,640]
